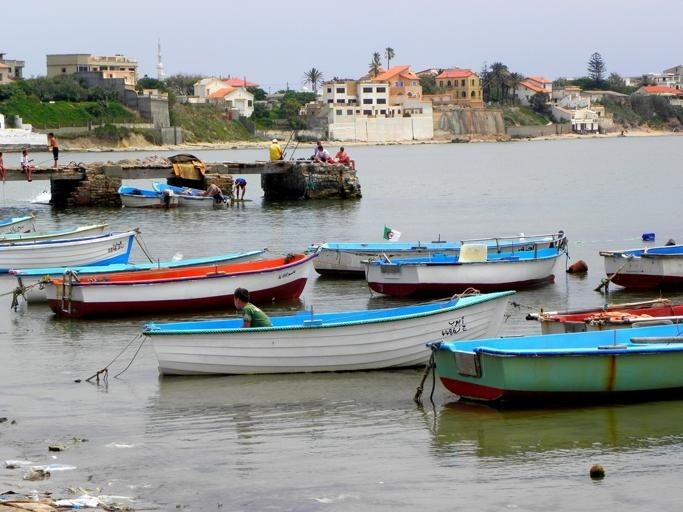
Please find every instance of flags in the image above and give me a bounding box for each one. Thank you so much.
[384,226,401,242]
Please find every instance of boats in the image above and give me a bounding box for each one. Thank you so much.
[7,247,270,304]
[420,323,683,402]
[0,227,143,273]
[359,246,565,297]
[152,180,232,208]
[523,296,683,336]
[40,249,321,316]
[114,184,179,208]
[136,289,516,377]
[599,244,683,290]
[0,212,37,236]
[0,223,111,243]
[302,231,570,277]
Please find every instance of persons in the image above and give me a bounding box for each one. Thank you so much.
[164,193,170,209]
[199,183,231,207]
[21,150,32,181]
[234,288,272,327]
[0,151,6,183]
[311,141,350,167]
[233,177,246,200]
[270,139,285,166]
[47,132,59,167]
